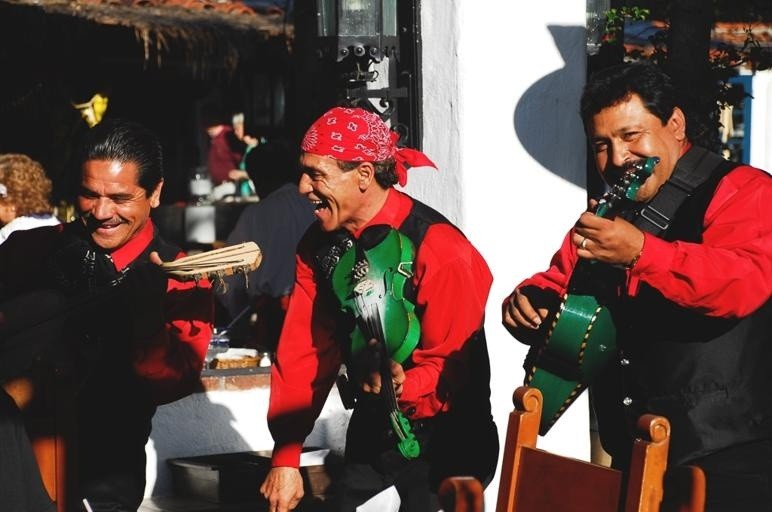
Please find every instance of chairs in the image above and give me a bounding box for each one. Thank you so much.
[492,386,671,512]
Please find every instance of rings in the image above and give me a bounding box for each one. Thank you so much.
[578,235,588,250]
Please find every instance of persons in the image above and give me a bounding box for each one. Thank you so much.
[258,105,501,511]
[500,62,771,511]
[149,105,318,347]
[0,117,216,512]
[0,153,60,244]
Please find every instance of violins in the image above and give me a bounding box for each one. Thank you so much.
[331,224,423,462]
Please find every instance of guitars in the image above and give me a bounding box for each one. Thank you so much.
[2,239,264,393]
[523,157,663,436]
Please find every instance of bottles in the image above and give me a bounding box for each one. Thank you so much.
[259,352,271,367]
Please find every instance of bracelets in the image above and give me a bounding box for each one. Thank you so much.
[623,248,643,271]
